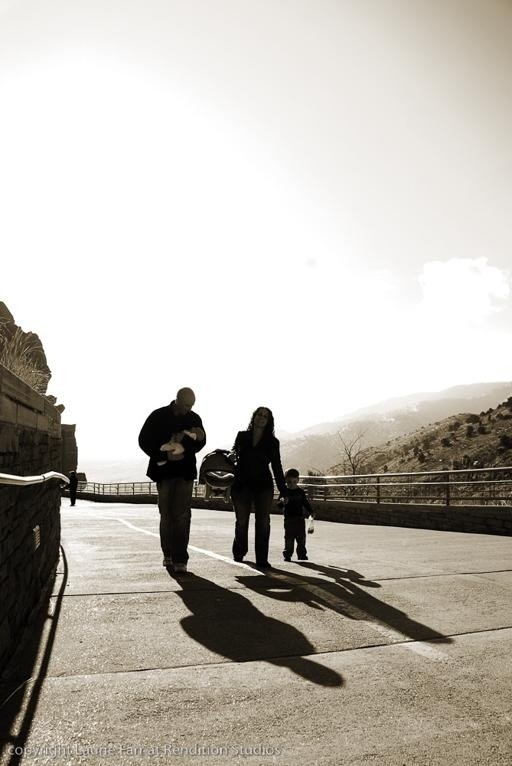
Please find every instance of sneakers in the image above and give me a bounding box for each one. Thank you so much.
[163,553,187,573]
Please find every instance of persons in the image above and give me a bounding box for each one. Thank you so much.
[229,407,287,568]
[278,467,314,561]
[138,387,206,575]
[65,471,78,506]
[156,426,205,466]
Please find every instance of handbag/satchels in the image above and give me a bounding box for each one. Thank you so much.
[240,465,274,501]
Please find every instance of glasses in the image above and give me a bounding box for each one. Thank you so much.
[257,414,267,418]
